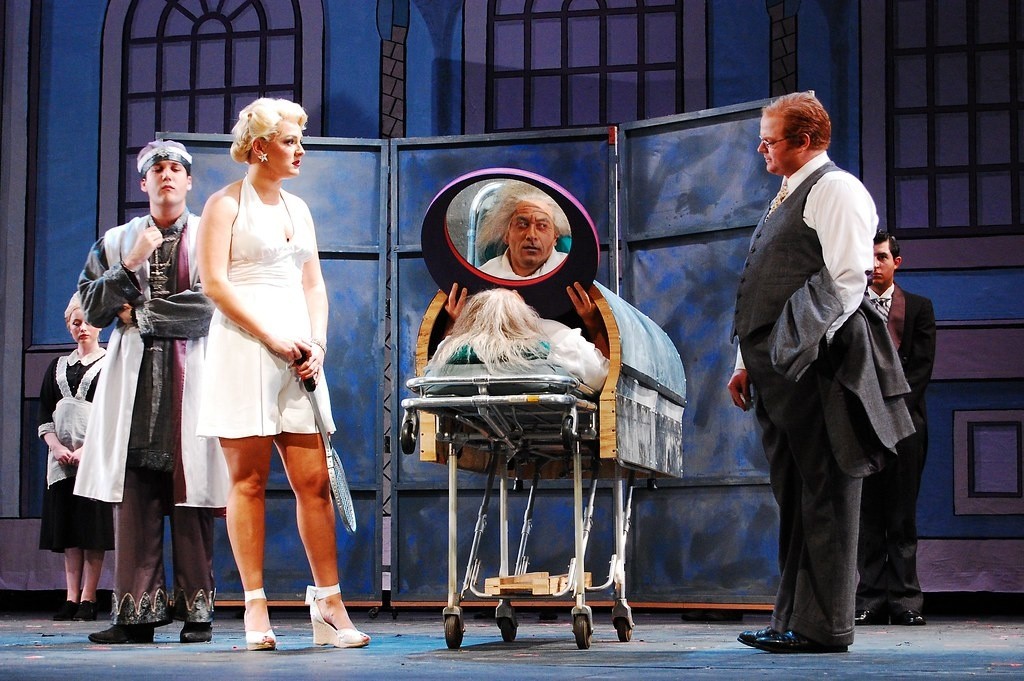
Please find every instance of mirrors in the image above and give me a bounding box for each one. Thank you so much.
[445,178,573,281]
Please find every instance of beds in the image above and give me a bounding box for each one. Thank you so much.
[400,167,687,649]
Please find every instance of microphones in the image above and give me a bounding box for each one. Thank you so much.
[294,349,316,392]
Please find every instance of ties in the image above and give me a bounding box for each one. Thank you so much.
[874,299,889,327]
[765,185,789,222]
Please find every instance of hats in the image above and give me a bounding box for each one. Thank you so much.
[136,139,192,177]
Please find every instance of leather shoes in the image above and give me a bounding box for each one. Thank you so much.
[754,630,848,653]
[88,625,155,643]
[855,611,889,625]
[736,625,780,649]
[891,609,925,625]
[179,622,212,643]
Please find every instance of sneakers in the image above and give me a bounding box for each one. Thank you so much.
[53,600,78,621]
[73,600,98,621]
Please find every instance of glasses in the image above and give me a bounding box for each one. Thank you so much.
[759,134,798,148]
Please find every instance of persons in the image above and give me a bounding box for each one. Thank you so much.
[427,281,610,390]
[77,138,214,647]
[854,228,937,626]
[197,98,371,651]
[728,90,879,654]
[474,179,572,281]
[36,291,106,623]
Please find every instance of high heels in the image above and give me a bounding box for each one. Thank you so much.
[305,584,372,648]
[244,589,277,650]
